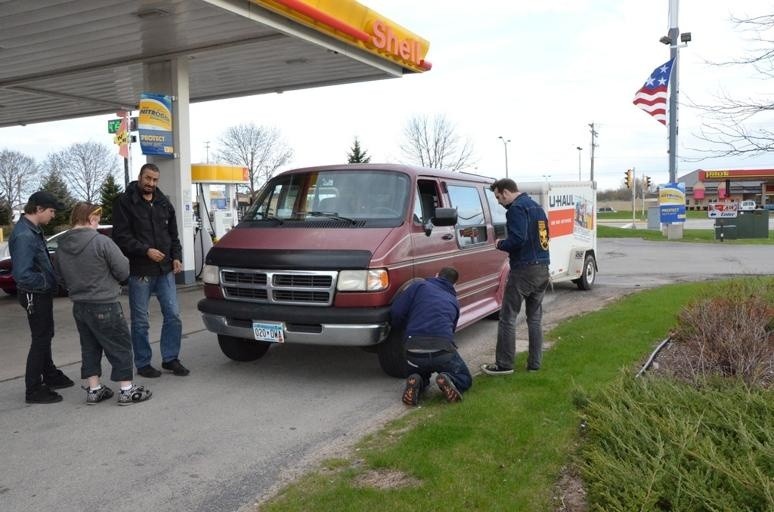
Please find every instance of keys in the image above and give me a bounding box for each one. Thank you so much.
[137,276,147,283]
[25,292,34,315]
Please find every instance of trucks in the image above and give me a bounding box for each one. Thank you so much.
[515,183,599,288]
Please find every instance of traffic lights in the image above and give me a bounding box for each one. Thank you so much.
[645,176,651,189]
[625,169,631,188]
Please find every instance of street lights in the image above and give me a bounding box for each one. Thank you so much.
[499,137,510,179]
[660,1,691,182]
[577,147,583,181]
[587,122,598,180]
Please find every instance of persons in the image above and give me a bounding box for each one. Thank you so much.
[392,268,472,406]
[480,178,552,374]
[113,164,191,378]
[9,190,74,404]
[54,201,152,407]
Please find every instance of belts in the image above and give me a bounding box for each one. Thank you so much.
[406,349,448,359]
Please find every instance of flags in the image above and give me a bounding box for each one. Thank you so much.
[632,56,676,126]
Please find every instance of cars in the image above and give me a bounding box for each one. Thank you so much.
[740,200,756,210]
[597,207,617,213]
[0,224,129,297]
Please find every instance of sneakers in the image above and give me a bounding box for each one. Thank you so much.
[480,363,514,375]
[436,375,463,402]
[403,374,423,405]
[526,363,539,373]
[86,384,114,405]
[137,365,161,378]
[117,384,154,406]
[162,359,189,376]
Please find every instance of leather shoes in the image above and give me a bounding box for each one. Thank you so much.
[43,371,73,389]
[25,391,62,403]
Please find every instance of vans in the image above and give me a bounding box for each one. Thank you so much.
[196,164,508,379]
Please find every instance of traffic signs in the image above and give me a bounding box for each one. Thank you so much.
[108,118,137,133]
